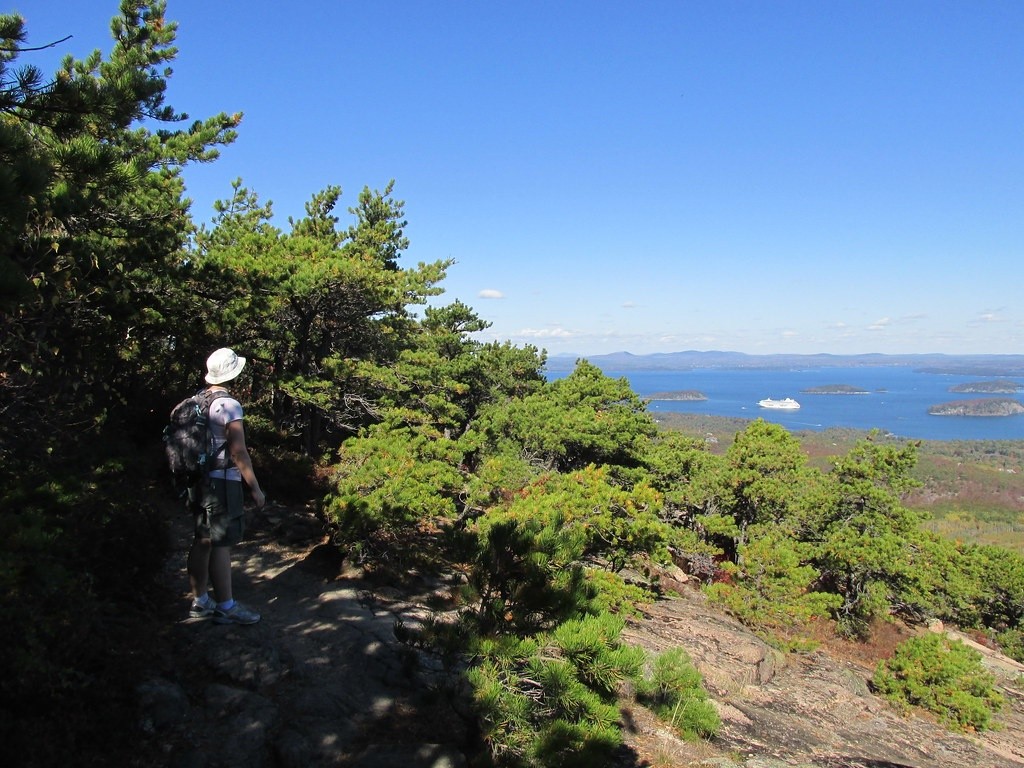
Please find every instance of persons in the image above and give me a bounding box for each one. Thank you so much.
[187,348,265,623]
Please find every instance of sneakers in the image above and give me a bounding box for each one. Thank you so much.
[212,600,261,624]
[189,597,217,618]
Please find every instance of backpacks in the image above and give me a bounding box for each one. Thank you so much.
[163,388,234,487]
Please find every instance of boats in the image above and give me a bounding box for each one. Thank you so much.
[756,399,800,409]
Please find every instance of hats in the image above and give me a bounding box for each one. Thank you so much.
[205,348,246,384]
